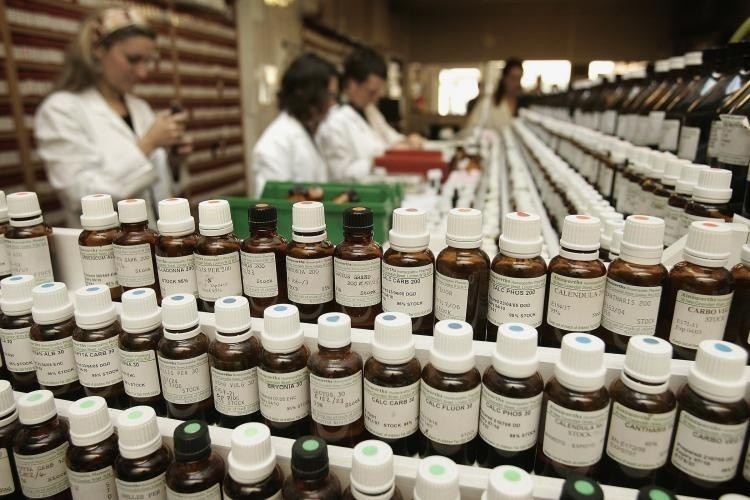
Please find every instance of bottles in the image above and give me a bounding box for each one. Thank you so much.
[167,100,195,161]
[0,183,750,500]
[398,50,750,247]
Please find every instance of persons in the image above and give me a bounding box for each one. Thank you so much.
[313,63,425,183]
[32,6,194,234]
[491,58,527,117]
[250,51,338,201]
[341,46,422,144]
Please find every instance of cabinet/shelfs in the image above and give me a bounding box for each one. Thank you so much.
[0,0,750,500]
